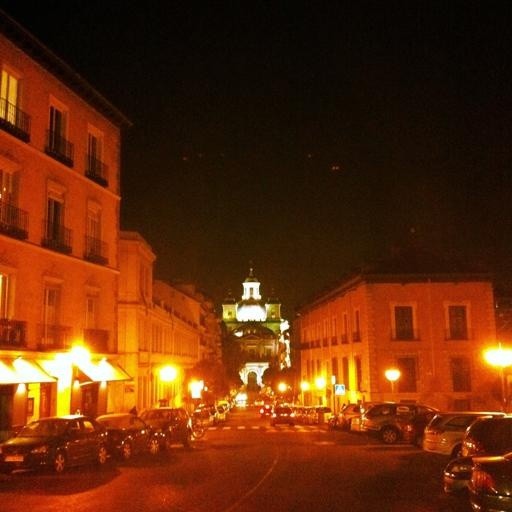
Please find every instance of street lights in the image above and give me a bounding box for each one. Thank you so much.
[489,339,511,413]
[384,363,404,403]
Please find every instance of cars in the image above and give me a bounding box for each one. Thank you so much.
[0,396,240,476]
[252,398,512,512]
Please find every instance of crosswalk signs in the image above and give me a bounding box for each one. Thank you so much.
[335,384,346,396]
